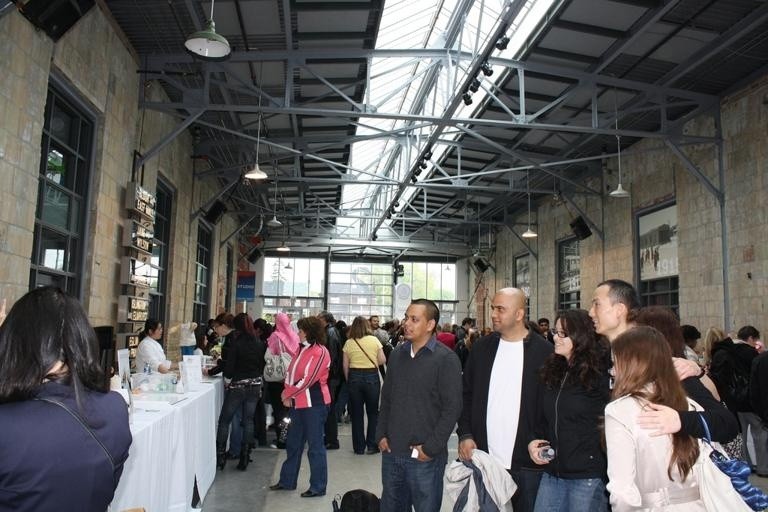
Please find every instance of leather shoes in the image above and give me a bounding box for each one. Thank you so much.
[270,484,297,490]
[300,489,328,498]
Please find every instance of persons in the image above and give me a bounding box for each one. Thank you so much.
[640,247,661,271]
[376,298,464,510]
[0,286,131,512]
[589,278,721,511]
[0,298,8,325]
[565,260,572,273]
[135,318,179,374]
[168,320,198,356]
[456,287,556,510]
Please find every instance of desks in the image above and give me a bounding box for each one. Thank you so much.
[100,355,225,512]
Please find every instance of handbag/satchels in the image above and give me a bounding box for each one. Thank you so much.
[695,439,768,512]
[263,352,295,384]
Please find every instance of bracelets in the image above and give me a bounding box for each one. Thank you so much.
[697,368,705,378]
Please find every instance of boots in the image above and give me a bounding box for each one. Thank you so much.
[235,440,252,471]
[216,440,226,472]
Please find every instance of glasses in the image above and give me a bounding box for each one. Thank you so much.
[550,327,572,339]
[213,323,223,330]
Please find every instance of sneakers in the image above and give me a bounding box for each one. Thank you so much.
[269,440,287,449]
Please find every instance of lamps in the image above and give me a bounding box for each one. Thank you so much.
[266,156,294,270]
[442,195,492,274]
[244,51,270,182]
[607,103,630,200]
[184,0,231,58]
[460,25,510,108]
[570,210,595,242]
[407,145,435,185]
[370,199,406,286]
[199,197,266,264]
[521,163,536,238]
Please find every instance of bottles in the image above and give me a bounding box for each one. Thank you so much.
[538,446,556,462]
[280,417,290,442]
[176,380,184,393]
[143,362,151,373]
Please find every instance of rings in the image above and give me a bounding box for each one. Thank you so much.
[458,454,462,457]
